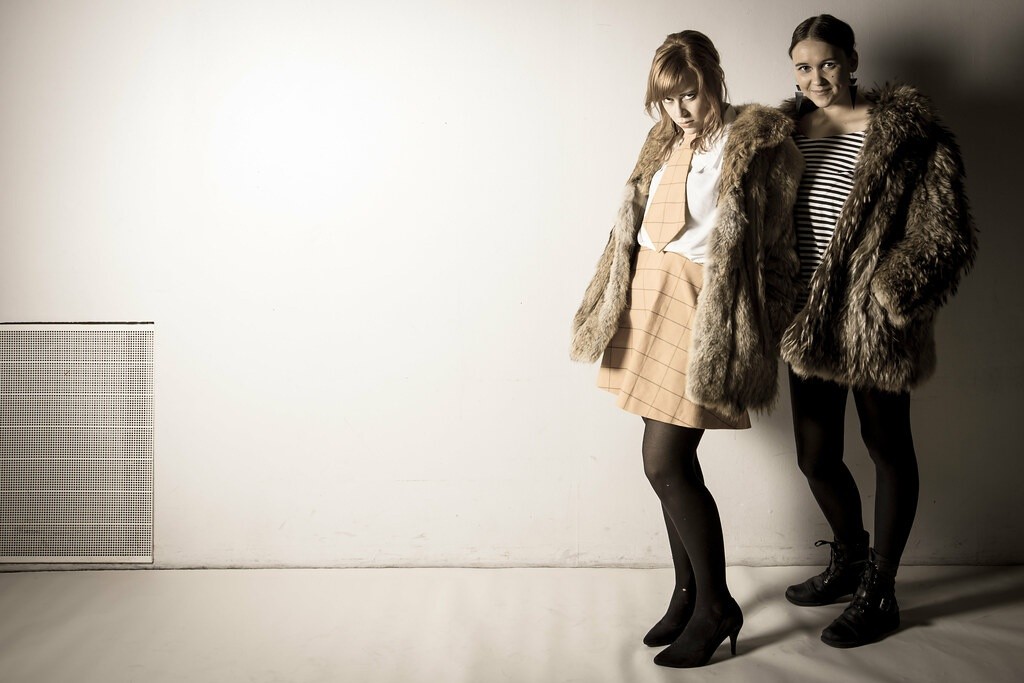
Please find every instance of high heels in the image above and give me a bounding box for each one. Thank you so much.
[642,612,689,647]
[654,598,744,668]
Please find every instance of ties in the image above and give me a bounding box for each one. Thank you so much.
[644,133,700,253]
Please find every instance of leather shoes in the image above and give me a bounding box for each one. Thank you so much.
[821,553,901,648]
[785,536,870,607]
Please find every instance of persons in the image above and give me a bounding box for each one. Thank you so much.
[770,14,980,648]
[567,30,805,669]
